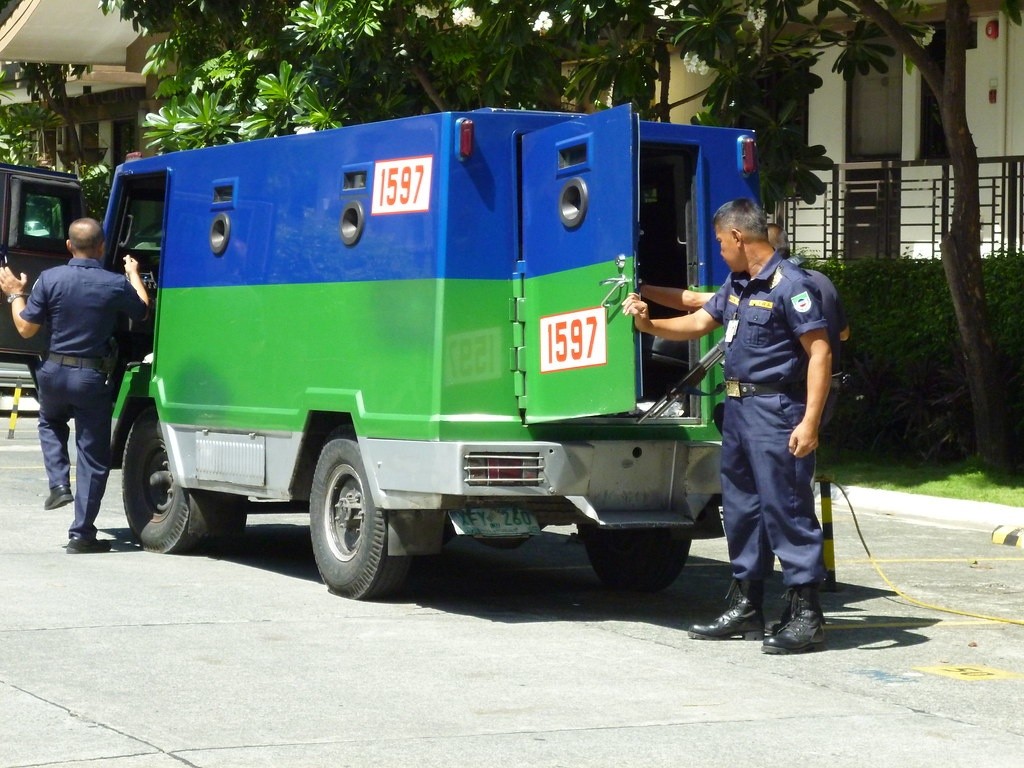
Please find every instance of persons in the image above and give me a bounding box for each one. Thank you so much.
[621,202,848,656]
[0,215,150,555]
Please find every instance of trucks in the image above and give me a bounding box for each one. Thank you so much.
[1,106,764,603]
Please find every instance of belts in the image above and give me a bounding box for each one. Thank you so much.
[725,380,786,398]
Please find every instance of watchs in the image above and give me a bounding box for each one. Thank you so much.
[6,292,22,303]
[637,276,644,288]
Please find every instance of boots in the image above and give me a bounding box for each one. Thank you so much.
[761,581,826,655]
[688,577,764,640]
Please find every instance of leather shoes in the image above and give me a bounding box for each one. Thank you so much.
[68,538,112,553]
[48,352,103,368]
[46,487,74,509]
